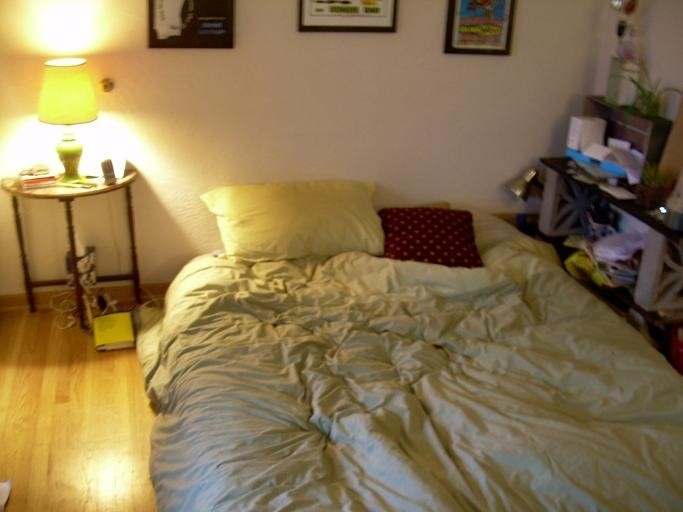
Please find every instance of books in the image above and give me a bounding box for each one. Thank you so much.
[93,312,135,351]
[584,242,640,287]
[20,174,57,189]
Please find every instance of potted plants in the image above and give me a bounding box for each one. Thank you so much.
[633,161,673,210]
[624,63,680,120]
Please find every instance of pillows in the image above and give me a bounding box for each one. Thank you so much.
[198,178,488,269]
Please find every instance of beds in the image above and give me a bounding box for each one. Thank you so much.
[162,230,682,512]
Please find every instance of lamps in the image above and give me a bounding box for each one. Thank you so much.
[35,56,98,184]
[505,164,544,205]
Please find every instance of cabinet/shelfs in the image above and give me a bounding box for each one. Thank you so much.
[586,95,673,169]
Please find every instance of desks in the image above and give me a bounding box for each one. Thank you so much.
[538,155,682,349]
[1,165,140,330]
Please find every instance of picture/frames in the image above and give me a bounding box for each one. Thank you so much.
[297,0,516,57]
